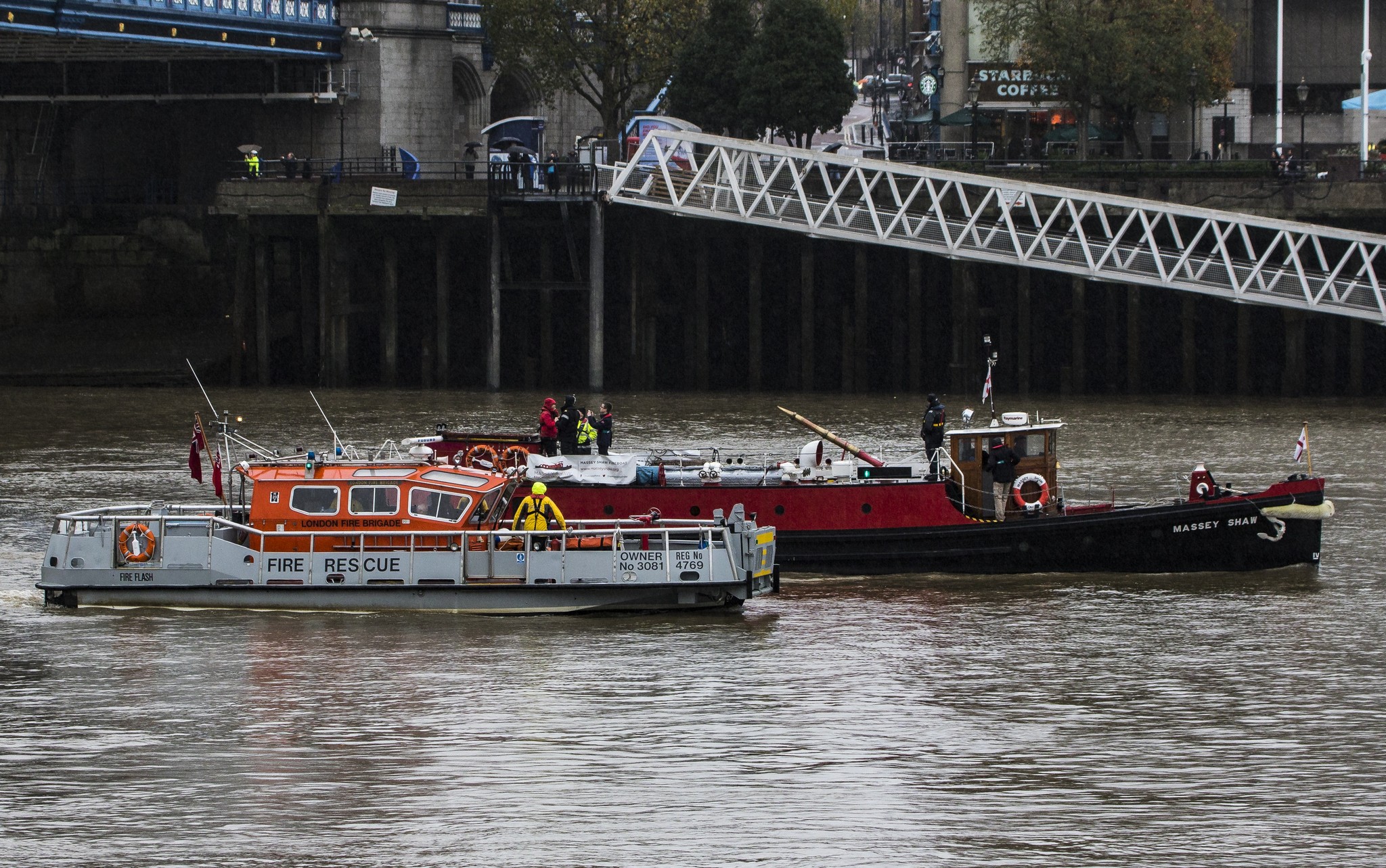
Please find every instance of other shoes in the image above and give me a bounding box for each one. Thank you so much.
[924,476,928,479]
[928,476,938,480]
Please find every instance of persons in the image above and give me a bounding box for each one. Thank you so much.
[920,393,946,479]
[538,394,613,457]
[1271,149,1298,183]
[242,139,591,196]
[511,482,567,550]
[982,365,991,404]
[984,438,1021,522]
[329,495,365,512]
[457,496,489,514]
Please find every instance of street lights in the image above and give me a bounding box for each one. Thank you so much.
[967,82,981,166]
[850,25,856,81]
[334,82,350,178]
[1296,76,1311,179]
[1189,62,1198,156]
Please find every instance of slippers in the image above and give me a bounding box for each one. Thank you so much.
[601,406,605,409]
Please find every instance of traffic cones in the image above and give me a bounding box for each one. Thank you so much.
[873,114,878,127]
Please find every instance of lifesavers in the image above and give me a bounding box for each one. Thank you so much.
[500,445,530,477]
[120,524,154,562]
[1013,473,1049,511]
[466,445,499,473]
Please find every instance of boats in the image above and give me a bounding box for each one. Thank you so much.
[35,357,778,618]
[385,331,1335,578]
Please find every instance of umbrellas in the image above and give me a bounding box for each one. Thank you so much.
[1341,88,1386,111]
[238,144,261,159]
[492,136,524,150]
[464,140,485,148]
[507,146,537,156]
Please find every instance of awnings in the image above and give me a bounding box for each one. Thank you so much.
[901,108,993,126]
[1045,125,1110,141]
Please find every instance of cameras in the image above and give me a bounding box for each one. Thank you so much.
[590,411,592,414]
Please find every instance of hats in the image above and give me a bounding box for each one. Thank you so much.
[927,394,936,402]
[577,407,586,415]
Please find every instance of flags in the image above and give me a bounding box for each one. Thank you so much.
[1294,427,1306,464]
[188,415,223,500]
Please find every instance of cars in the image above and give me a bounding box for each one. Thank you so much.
[859,73,913,100]
